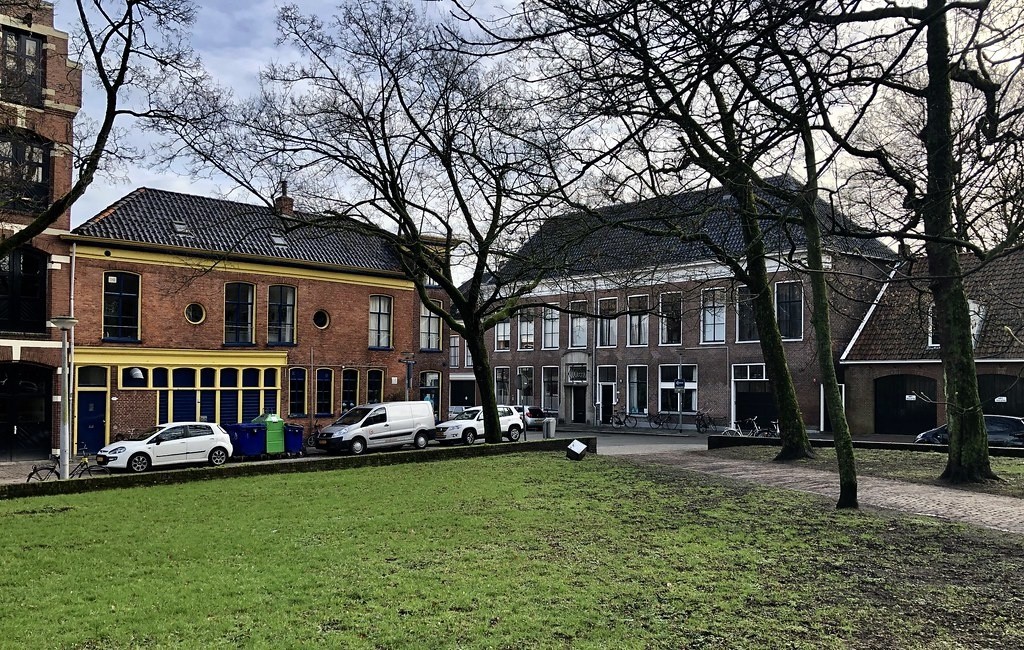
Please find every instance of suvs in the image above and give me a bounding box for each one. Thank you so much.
[435,405,525,444]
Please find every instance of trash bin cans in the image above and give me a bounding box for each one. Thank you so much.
[284,423,304,458]
[542,417,556,439]
[223,424,267,461]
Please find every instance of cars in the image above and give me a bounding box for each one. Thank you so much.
[96,422,234,473]
[915,414,1024,448]
[514,405,546,430]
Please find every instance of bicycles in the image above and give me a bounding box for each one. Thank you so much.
[698,408,717,434]
[694,407,703,432]
[610,405,638,430]
[25,441,111,483]
[757,421,780,437]
[649,409,678,430]
[722,415,772,437]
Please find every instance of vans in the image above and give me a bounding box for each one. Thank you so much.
[318,401,438,455]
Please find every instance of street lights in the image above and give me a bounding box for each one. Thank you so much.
[51,314,79,482]
[400,352,414,401]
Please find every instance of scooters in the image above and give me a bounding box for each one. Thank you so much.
[307,419,324,448]
